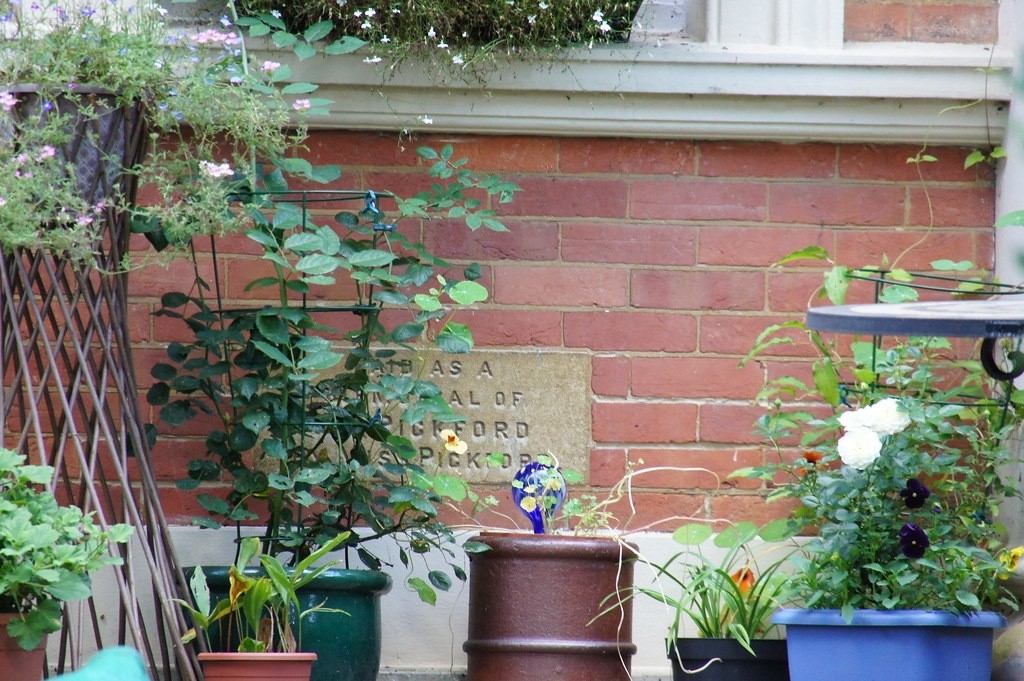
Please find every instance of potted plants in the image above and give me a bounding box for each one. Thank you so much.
[584,533,815,681]
[0,446,137,681]
[163,530,353,681]
[125,16,523,681]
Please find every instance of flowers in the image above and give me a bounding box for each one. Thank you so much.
[707,26,1024,615]
[0,0,312,275]
[394,277,735,681]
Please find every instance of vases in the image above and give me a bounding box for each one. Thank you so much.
[768,606,1006,681]
[0,82,132,222]
[461,533,638,681]
